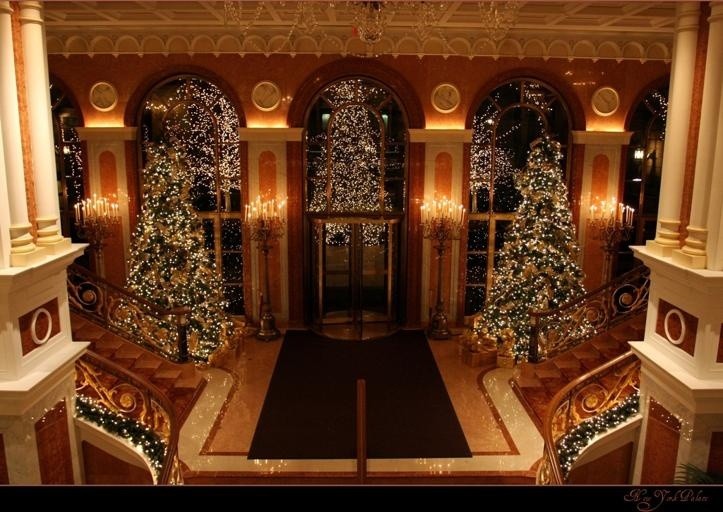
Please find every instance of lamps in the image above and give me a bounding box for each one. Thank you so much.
[224,0,523,59]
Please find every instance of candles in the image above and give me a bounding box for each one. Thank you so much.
[588,195,635,227]
[244,195,287,224]
[418,196,467,225]
[74,192,118,225]
